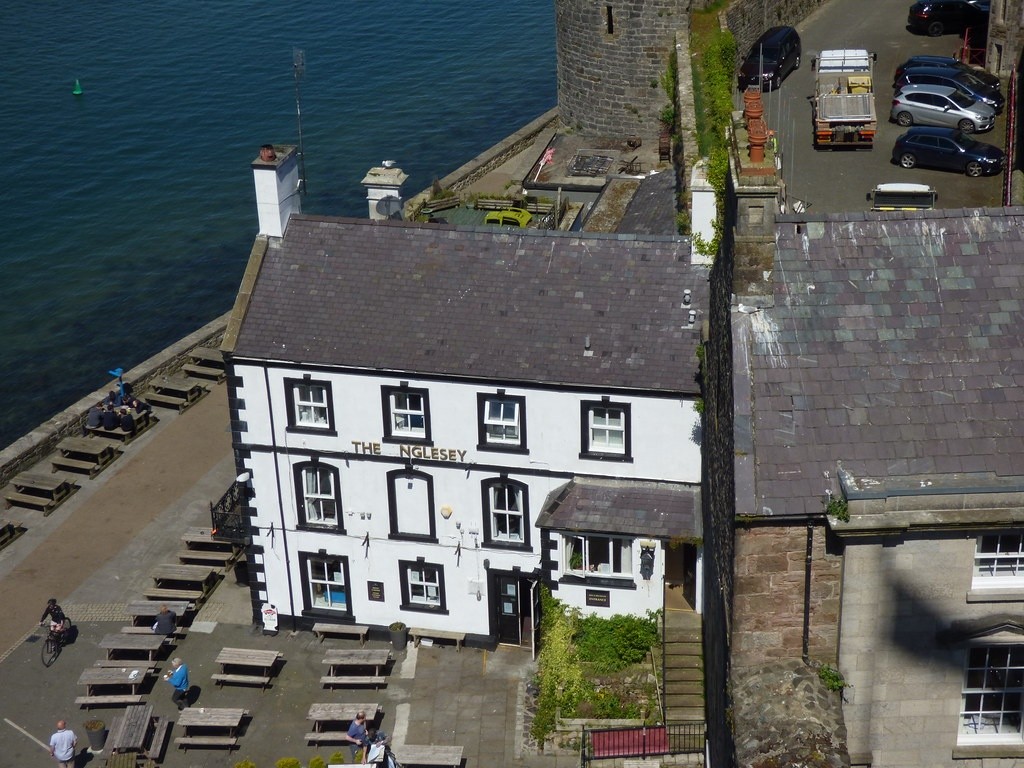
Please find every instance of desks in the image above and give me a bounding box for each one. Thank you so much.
[322,649,391,677]
[390,745,464,768]
[187,347,225,368]
[306,703,378,733]
[181,527,232,546]
[147,564,215,592]
[215,647,280,677]
[77,668,148,697]
[102,404,147,435]
[55,437,111,467]
[148,375,199,404]
[114,705,157,752]
[177,708,245,737]
[9,472,66,505]
[98,633,167,661]
[126,600,190,627]
[106,752,136,768]
[0,518,15,551]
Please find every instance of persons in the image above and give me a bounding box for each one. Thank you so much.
[119,409,134,432]
[345,711,368,764]
[103,391,151,431]
[154,605,177,637]
[49,720,79,768]
[87,402,104,429]
[362,728,402,768]
[102,405,120,431]
[39,599,65,651]
[162,658,189,713]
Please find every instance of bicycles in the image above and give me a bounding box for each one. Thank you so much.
[38,617,74,668]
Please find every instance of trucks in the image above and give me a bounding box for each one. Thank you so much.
[866,182,939,211]
[811,49,878,146]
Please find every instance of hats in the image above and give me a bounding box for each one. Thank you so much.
[48,598,56,605]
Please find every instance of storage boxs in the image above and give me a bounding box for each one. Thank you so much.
[848,76,871,94]
[421,638,433,646]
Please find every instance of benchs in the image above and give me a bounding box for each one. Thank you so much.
[101,716,124,760]
[305,732,346,750]
[143,587,205,609]
[65,477,78,495]
[85,424,132,445]
[48,457,97,480]
[408,627,466,653]
[274,653,284,669]
[161,638,173,652]
[185,603,195,615]
[94,660,159,676]
[74,695,142,712]
[211,673,270,693]
[3,491,52,517]
[242,709,250,721]
[320,676,386,693]
[144,393,186,415]
[108,441,124,458]
[464,633,498,651]
[145,669,155,673]
[9,520,26,538]
[148,719,168,758]
[196,382,210,397]
[377,706,383,716]
[176,550,233,571]
[174,737,238,756]
[211,568,223,585]
[121,627,183,642]
[387,652,393,664]
[312,623,370,648]
[181,364,224,385]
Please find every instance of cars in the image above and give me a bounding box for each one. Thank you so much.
[737,26,802,93]
[907,0,990,37]
[892,126,1006,177]
[894,54,1001,91]
[894,67,1006,116]
[890,84,997,134]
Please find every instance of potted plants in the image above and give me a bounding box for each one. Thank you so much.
[388,620,407,651]
[568,552,582,570]
[82,720,106,751]
